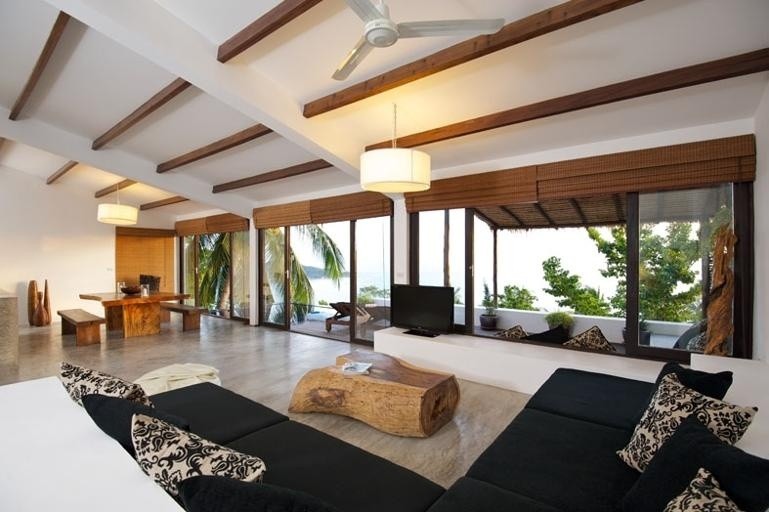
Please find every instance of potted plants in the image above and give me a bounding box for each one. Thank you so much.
[621,318,651,346]
[545,311,575,338]
[480,297,499,331]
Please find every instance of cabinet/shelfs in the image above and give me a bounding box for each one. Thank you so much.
[374,327,690,396]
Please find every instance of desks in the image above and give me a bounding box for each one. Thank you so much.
[79,291,193,338]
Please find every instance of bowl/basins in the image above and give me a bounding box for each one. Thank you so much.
[120,288,140,294]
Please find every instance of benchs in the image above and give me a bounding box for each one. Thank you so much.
[57,309,109,346]
[160,302,208,332]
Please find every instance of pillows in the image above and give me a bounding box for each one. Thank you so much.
[492,324,617,354]
[175,471,335,511]
[125,411,270,496]
[80,391,158,459]
[58,361,153,407]
[611,360,769,512]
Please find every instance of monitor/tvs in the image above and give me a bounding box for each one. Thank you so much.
[390,284,454,338]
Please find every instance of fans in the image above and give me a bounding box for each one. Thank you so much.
[331,0,505,82]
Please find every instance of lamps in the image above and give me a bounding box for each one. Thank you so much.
[97,177,137,225]
[360,104,431,193]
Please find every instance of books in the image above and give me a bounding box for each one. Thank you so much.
[343,362,373,375]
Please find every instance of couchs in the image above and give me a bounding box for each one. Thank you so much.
[0,355,769,512]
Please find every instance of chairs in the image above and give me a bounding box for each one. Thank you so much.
[326,302,391,337]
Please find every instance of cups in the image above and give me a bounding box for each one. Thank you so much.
[141,284,149,298]
